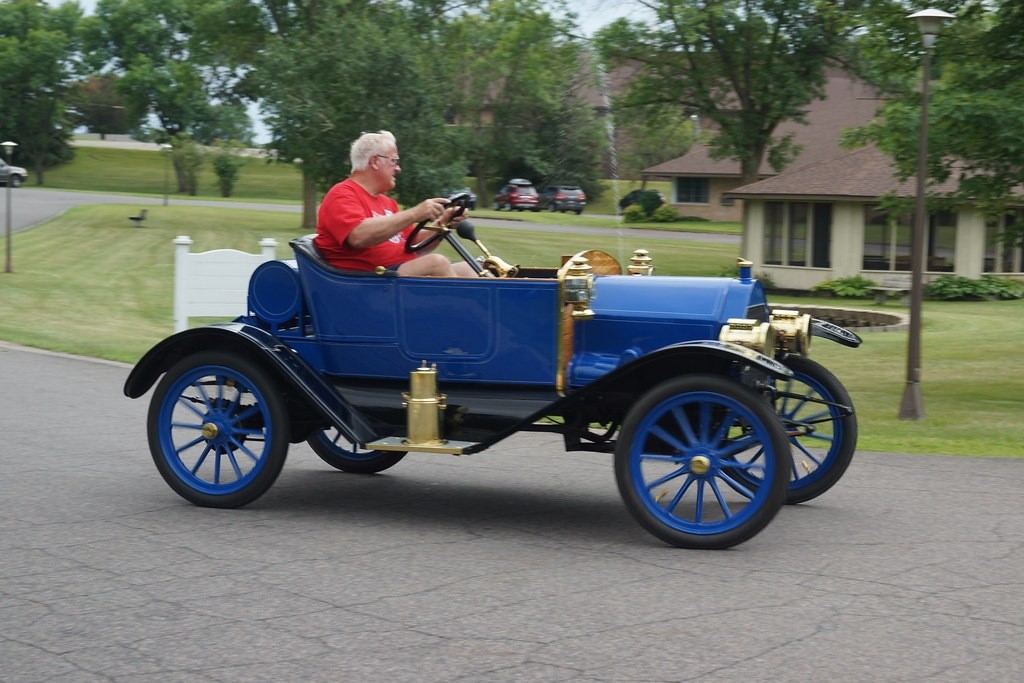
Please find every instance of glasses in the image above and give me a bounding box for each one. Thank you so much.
[373,152,400,166]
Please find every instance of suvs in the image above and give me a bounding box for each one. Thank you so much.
[492,184,538,212]
[537,184,587,215]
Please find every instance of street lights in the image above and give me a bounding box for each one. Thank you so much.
[896,8,959,422]
[0,141,19,270]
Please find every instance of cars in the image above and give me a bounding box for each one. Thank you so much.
[442,187,478,211]
[0,158,28,189]
[122,191,864,551]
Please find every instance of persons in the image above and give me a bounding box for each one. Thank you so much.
[313,131,478,277]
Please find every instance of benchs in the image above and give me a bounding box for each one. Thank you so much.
[864,281,912,306]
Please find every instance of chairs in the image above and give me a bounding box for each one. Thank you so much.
[289,232,401,278]
